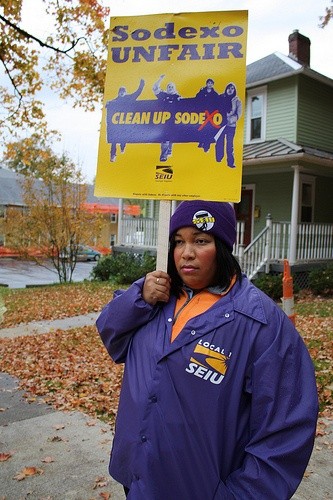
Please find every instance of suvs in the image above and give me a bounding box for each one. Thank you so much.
[59,244,100,262]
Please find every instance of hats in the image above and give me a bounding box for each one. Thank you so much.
[169,199,238,248]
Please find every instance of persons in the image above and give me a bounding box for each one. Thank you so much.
[93,198,320,500]
[215,84,241,168]
[151,74,181,162]
[192,78,218,151]
[105,79,145,163]
